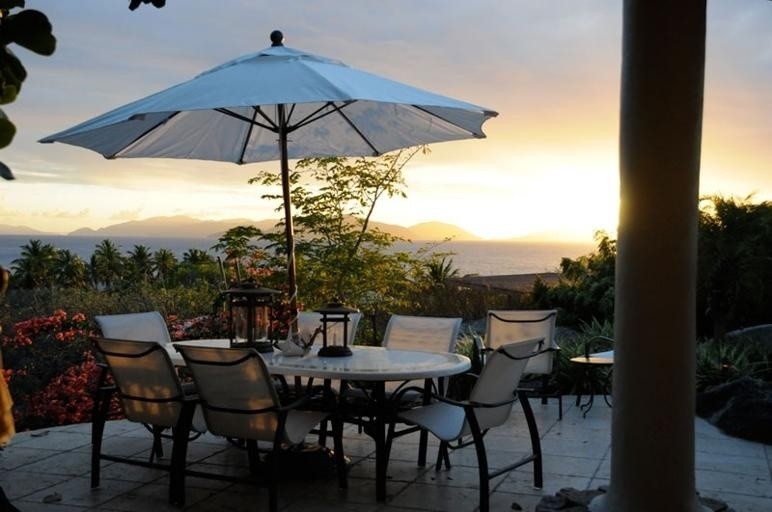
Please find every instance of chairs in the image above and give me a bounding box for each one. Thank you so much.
[469,310,561,406]
[280,310,364,459]
[382,338,545,512]
[173,342,349,497]
[87,335,245,501]
[341,313,464,474]
[90,311,186,458]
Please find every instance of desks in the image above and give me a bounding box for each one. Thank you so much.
[163,337,471,507]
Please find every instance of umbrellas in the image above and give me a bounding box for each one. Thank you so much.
[35,28,500,343]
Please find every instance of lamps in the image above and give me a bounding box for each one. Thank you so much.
[311,296,359,358]
[219,276,283,354]
